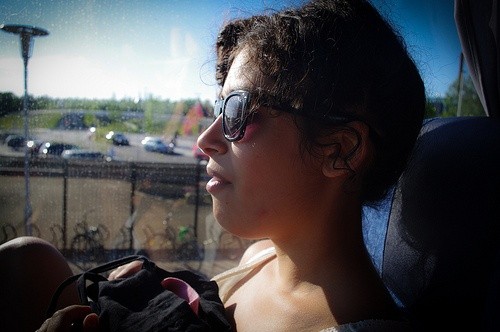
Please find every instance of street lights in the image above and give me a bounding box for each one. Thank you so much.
[0,21,54,239]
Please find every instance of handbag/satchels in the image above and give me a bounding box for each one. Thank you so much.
[49,254,235,332]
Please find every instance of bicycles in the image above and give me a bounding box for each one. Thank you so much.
[71,209,244,274]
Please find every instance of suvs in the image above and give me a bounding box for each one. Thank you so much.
[2,132,107,163]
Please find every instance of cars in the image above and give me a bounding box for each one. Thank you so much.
[106,130,129,146]
[140,137,174,154]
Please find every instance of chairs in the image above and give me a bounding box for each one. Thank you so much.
[356,114,500,332]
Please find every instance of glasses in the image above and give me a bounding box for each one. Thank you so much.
[213,88,350,142]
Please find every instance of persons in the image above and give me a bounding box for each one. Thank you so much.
[0,1,429,332]
